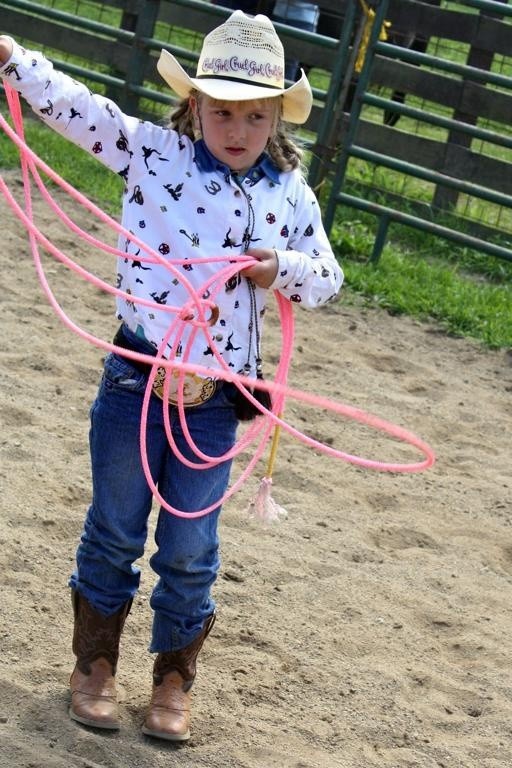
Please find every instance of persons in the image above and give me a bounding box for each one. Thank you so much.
[273,0,321,81]
[1,9,344,742]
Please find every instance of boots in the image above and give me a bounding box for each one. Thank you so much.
[70,586,132,729]
[141,615,216,741]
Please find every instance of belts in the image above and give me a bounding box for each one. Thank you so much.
[114,330,218,409]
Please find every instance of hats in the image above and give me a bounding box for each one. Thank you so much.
[156,9,313,125]
